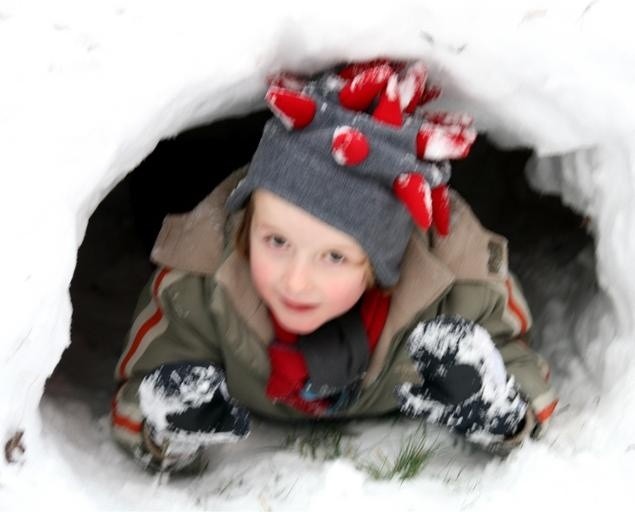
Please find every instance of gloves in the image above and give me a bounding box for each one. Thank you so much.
[139,362,250,461]
[394,317,528,453]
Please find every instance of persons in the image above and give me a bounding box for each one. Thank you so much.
[107,52,565,487]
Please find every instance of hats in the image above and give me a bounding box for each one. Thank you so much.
[223,62,478,286]
